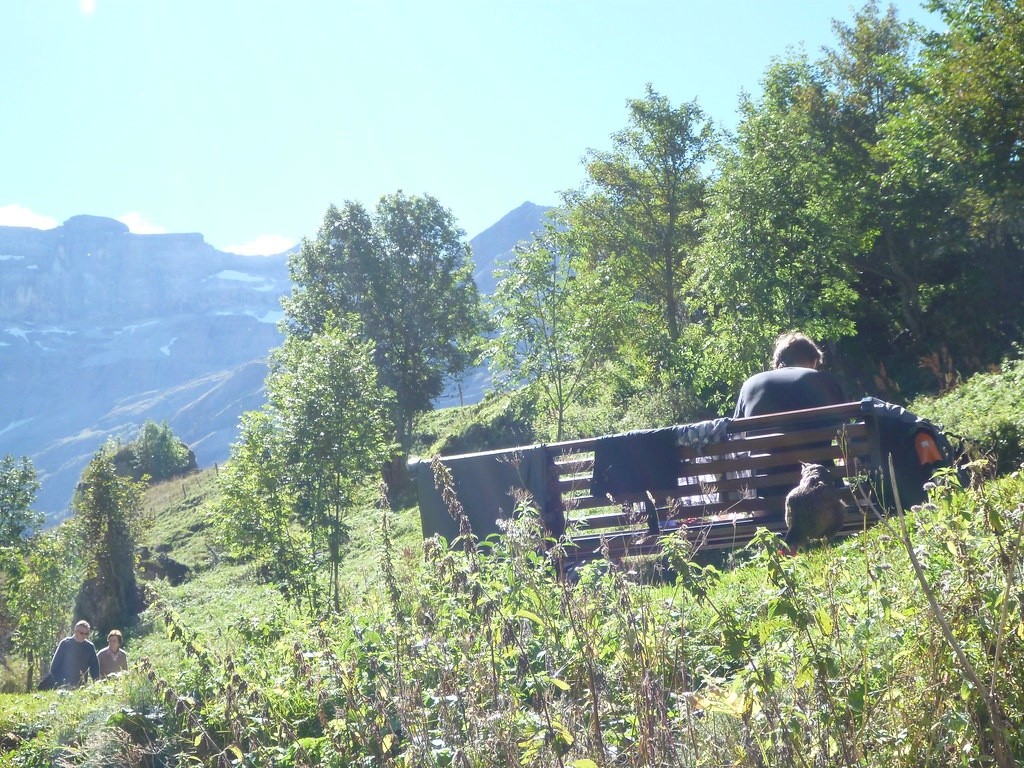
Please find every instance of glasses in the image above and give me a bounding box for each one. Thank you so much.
[76,630,90,636]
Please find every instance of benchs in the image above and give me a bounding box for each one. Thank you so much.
[544,400,885,579]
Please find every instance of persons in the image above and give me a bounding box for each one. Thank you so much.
[50,620,100,689]
[97,630,127,681]
[731,334,846,515]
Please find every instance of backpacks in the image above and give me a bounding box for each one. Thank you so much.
[894,422,968,506]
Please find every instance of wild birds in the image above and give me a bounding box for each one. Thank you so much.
[778,460,839,559]
